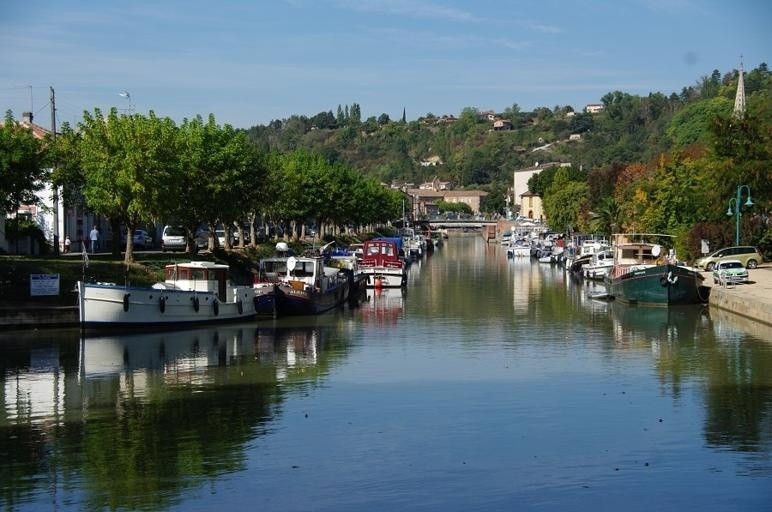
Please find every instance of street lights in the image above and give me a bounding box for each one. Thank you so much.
[726,184,756,245]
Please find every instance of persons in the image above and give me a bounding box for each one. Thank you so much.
[89,225,100,255]
[63,234,72,253]
[748,208,771,237]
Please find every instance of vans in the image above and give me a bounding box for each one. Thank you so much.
[693,245,766,271]
[158,220,267,253]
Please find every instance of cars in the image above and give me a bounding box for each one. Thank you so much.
[122,229,153,249]
[446,211,531,221]
[713,259,749,285]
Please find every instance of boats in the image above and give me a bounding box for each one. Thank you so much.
[498,221,709,311]
[72,258,278,329]
[262,197,449,314]
[71,318,260,387]
